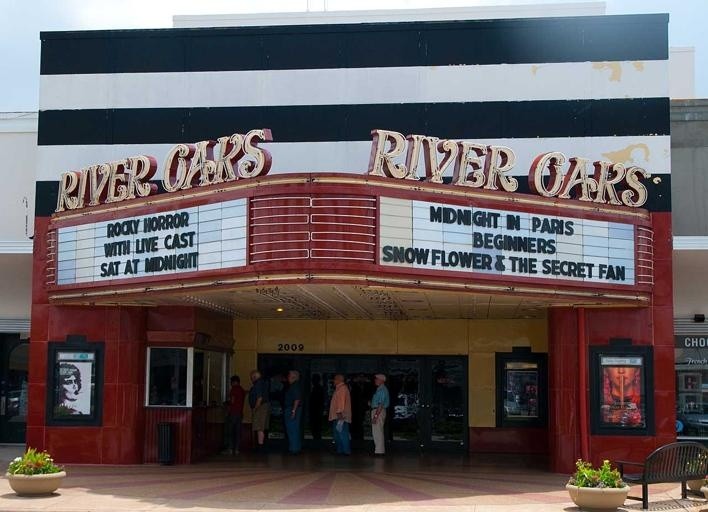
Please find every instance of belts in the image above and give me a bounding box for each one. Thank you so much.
[371,407,377,411]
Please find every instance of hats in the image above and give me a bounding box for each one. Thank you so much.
[374,374,387,382]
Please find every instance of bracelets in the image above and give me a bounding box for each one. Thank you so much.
[291,407,296,415]
[254,407,257,410]
[373,414,378,418]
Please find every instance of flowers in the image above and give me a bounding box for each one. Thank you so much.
[681,453,708,481]
[4,446,65,476]
[561,457,627,499]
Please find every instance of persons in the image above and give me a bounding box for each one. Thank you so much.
[308,372,326,441]
[602,366,642,425]
[367,373,389,457]
[55,362,83,415]
[244,370,271,456]
[284,370,302,457]
[327,373,352,455]
[218,374,246,456]
[347,373,365,451]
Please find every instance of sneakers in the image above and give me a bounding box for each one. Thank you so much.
[218,448,240,457]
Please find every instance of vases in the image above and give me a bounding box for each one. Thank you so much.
[685,480,707,493]
[4,471,67,497]
[564,486,631,510]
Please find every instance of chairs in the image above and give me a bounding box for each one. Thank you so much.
[616,440,707,509]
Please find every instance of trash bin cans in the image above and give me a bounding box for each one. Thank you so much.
[158,422,176,465]
